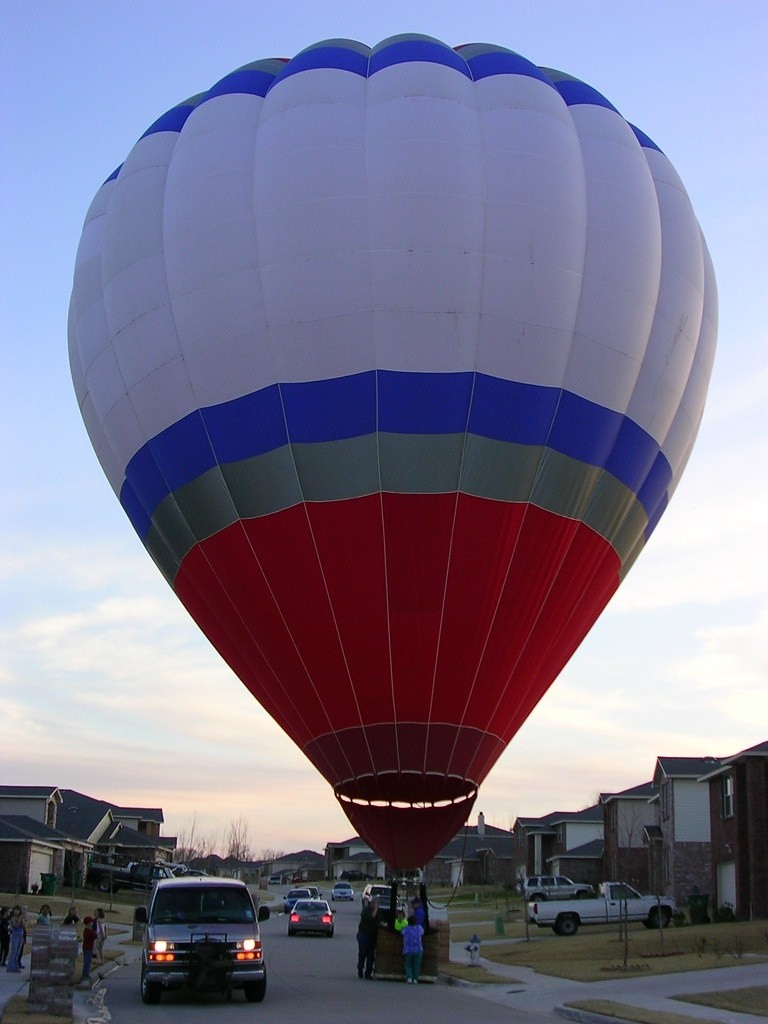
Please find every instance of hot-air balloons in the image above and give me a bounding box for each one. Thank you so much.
[70,32,720,983]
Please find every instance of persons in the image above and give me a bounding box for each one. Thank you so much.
[35,904,53,927]
[356,895,381,981]
[80,916,99,980]
[91,907,109,966]
[400,915,425,984]
[61,907,80,929]
[0,905,28,973]
[395,910,408,933]
[410,897,426,928]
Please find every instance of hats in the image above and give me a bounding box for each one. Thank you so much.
[83,916,93,924]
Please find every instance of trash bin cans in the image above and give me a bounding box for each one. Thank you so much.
[40,873,57,895]
[686,894,711,925]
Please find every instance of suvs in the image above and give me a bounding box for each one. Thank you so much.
[521,873,595,903]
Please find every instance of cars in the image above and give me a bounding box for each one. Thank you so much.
[267,876,282,884]
[283,888,314,912]
[340,870,371,881]
[331,880,354,902]
[288,899,337,937]
[363,884,401,909]
[299,886,322,900]
[290,876,317,884]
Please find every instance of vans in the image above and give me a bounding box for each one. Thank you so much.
[134,874,271,1004]
[393,867,422,886]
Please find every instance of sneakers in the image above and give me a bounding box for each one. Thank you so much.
[407,978,417,984]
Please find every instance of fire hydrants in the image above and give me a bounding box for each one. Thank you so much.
[464,932,482,968]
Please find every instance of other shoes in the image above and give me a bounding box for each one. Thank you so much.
[6,969,21,973]
[19,965,25,969]
[365,974,373,980]
[358,970,363,978]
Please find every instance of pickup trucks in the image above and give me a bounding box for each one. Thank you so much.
[87,861,177,894]
[527,881,677,935]
